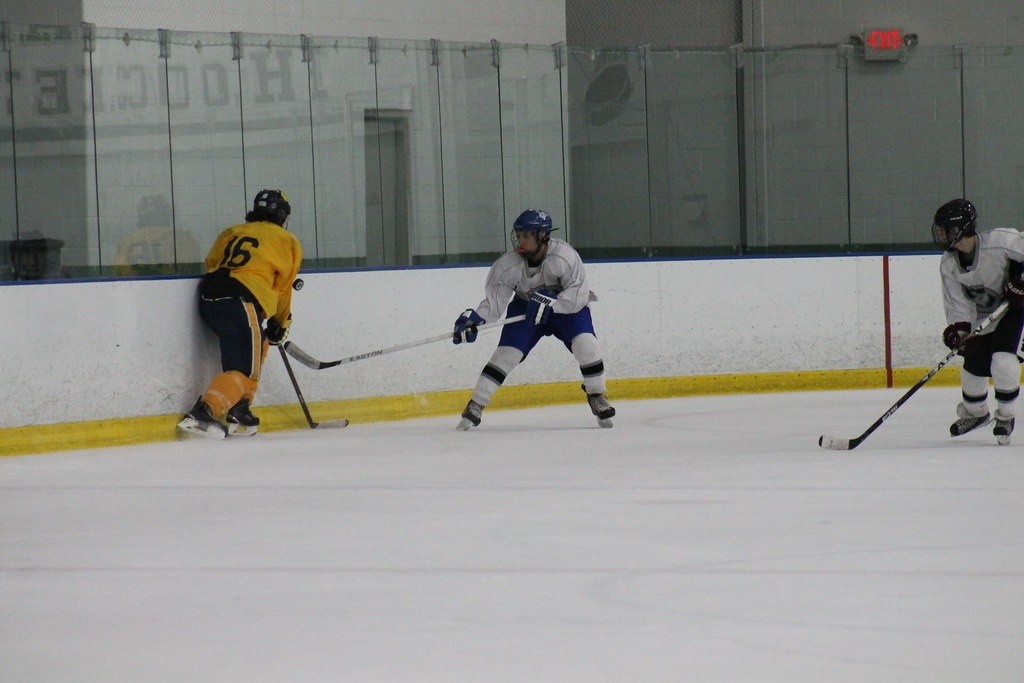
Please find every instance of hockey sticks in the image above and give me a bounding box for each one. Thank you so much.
[283,313,526,371]
[277,344,349,429]
[818,300,1008,450]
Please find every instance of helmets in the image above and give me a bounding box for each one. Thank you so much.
[935,199,979,226]
[512,208,553,233]
[253,189,292,215]
[132,195,171,225]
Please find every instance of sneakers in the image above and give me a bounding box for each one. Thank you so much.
[176,395,229,440]
[456,399,485,433]
[991,410,1017,445]
[224,397,260,438]
[949,403,992,440]
[585,394,617,428]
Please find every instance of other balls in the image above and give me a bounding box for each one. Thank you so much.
[292,278,304,291]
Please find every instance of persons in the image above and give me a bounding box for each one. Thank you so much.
[931,199,1024,445]
[112,193,201,278]
[8,229,72,281]
[175,190,303,439]
[453,209,616,431]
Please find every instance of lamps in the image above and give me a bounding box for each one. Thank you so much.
[850,29,919,62]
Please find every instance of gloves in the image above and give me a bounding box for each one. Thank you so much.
[527,287,559,329]
[453,308,486,344]
[1005,275,1024,307]
[264,315,292,346]
[942,322,974,356]
